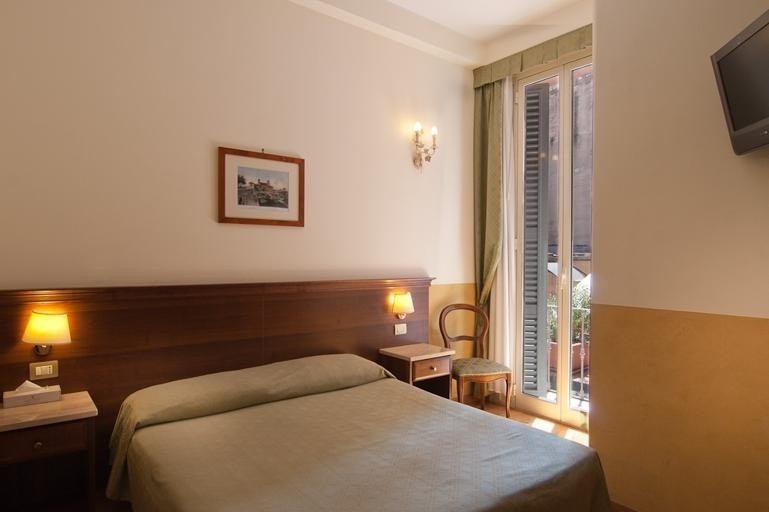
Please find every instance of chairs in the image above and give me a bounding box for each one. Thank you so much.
[439,304,513,419]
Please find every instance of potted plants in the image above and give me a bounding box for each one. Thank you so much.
[549,284,589,376]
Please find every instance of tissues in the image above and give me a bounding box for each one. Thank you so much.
[3,378,65,410]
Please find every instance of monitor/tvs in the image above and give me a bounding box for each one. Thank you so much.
[710,10,769,155]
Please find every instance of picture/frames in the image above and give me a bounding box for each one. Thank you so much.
[218,146,304,227]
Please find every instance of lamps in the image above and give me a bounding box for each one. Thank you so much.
[392,292,414,319]
[21,307,71,355]
[412,121,439,168]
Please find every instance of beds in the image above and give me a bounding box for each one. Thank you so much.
[123,353,597,512]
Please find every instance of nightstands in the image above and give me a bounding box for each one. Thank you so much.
[378,342,456,400]
[0,391,99,511]
